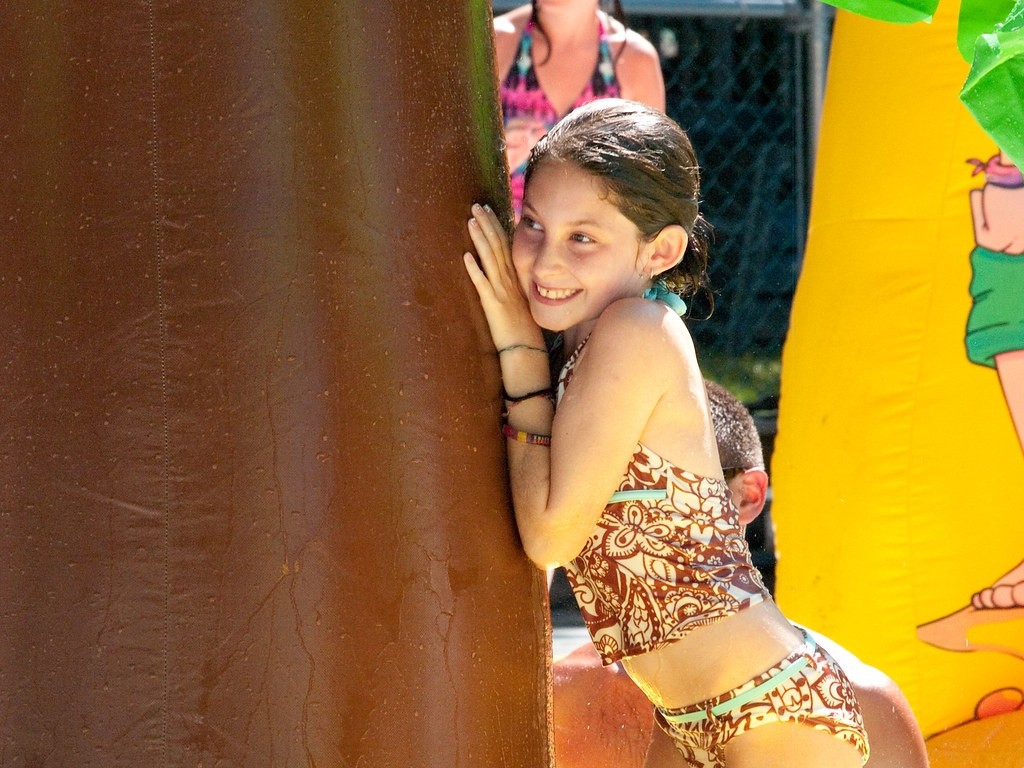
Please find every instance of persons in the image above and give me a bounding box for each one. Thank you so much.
[487,1,667,243]
[551,377,932,768]
[459,96,870,768]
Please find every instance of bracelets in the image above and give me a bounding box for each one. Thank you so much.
[501,423,551,447]
[501,382,555,402]
[494,344,551,358]
